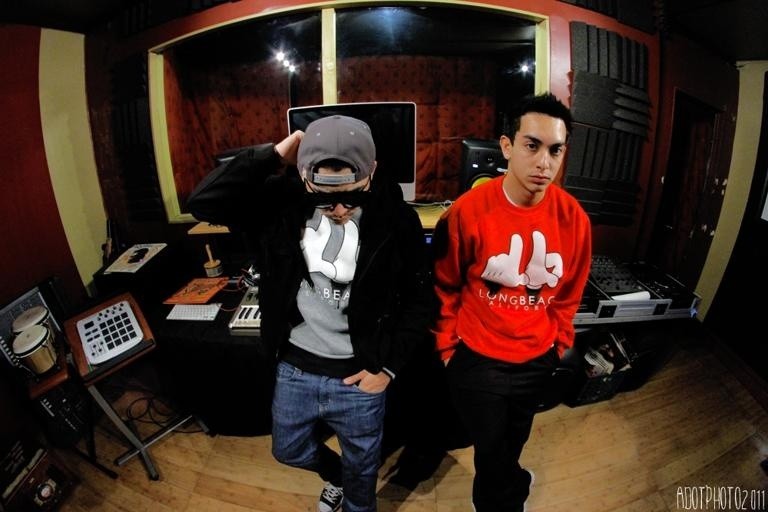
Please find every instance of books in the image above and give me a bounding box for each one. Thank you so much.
[161,276,229,305]
[105,242,167,274]
[581,329,636,378]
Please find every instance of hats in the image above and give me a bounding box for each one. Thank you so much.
[298,114,377,184]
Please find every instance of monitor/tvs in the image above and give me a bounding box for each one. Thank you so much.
[287,100,417,201]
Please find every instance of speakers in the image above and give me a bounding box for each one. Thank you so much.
[457,140,508,197]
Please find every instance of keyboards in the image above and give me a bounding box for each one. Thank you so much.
[165,303,222,321]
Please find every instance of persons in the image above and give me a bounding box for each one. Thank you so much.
[184,110,441,511]
[388,92,594,511]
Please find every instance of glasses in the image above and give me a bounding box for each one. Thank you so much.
[307,190,372,209]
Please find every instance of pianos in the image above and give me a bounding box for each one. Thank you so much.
[227,285,261,339]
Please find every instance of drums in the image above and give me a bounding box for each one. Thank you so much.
[13,325,61,381]
[9,304,56,345]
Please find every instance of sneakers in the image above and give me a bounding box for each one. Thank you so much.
[319,482,344,512]
[521,468,534,512]
